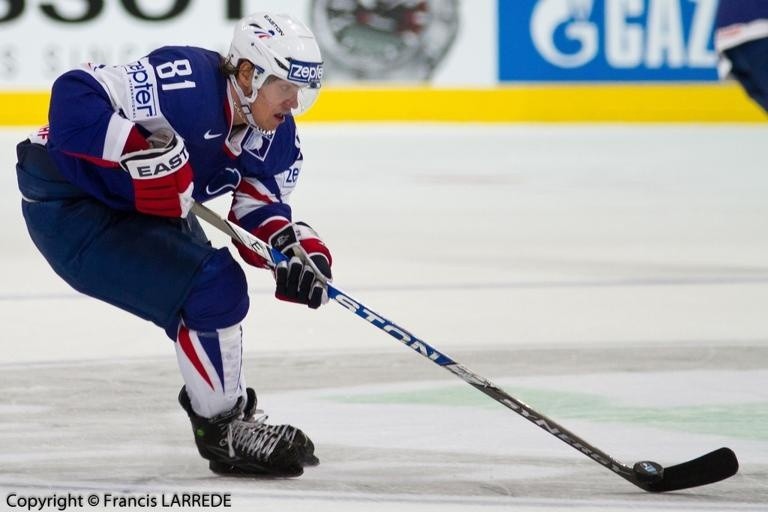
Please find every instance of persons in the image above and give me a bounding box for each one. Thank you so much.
[715,0,766,115]
[15,11,334,470]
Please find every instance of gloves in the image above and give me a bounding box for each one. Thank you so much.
[268,221,332,309]
[121,129,195,219]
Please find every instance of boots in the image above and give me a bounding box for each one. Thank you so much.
[177,386,301,472]
[246,384,314,461]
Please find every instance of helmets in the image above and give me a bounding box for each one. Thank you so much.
[225,11,325,89]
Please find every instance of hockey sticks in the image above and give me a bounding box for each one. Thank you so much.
[190,202,739,492]
